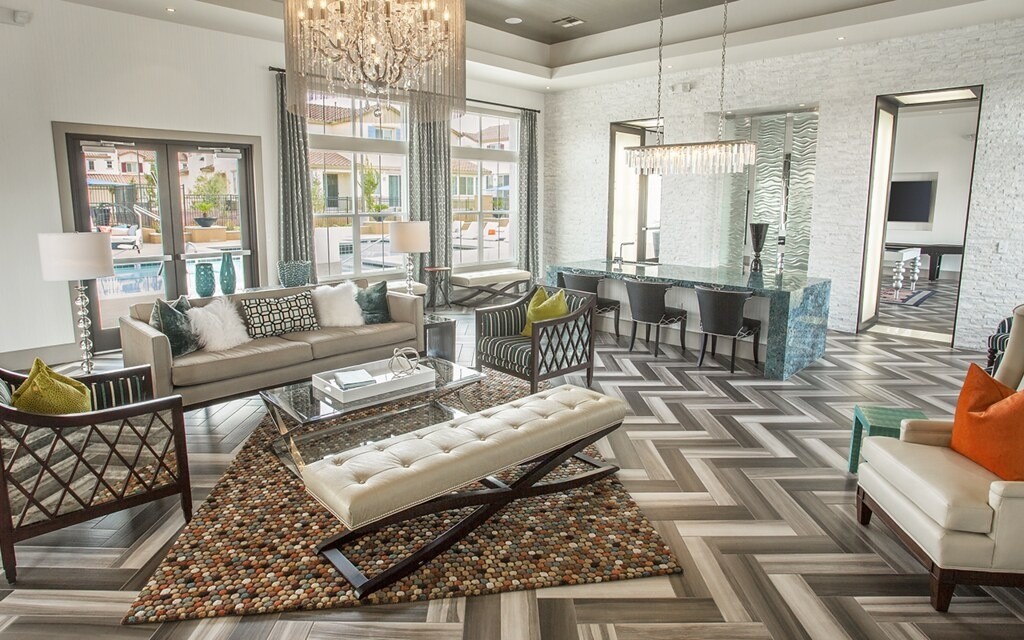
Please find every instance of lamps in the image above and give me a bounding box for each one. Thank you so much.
[624,0,758,175]
[283,0,467,124]
[38,233,115,376]
[389,221,429,295]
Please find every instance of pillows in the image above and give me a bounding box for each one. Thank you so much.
[950,362,1024,481]
[149,279,395,359]
[522,286,571,336]
[10,357,93,414]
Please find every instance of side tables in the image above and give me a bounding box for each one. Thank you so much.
[424,314,457,363]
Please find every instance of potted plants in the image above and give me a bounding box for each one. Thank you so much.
[194,202,218,227]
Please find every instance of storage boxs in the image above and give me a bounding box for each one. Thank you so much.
[312,357,435,404]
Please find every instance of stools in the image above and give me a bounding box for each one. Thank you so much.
[849,404,928,473]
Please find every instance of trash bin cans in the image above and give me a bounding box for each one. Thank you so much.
[423,316,456,362]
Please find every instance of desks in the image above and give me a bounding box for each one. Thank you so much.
[424,267,451,311]
[546,261,831,382]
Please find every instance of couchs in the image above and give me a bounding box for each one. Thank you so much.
[118,278,425,408]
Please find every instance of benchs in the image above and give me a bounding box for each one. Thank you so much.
[452,268,531,307]
[302,385,627,598]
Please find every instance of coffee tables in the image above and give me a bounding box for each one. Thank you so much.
[259,355,487,481]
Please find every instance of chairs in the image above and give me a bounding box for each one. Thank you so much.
[857,302,1024,615]
[475,273,762,395]
[0,363,192,584]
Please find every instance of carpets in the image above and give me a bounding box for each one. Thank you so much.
[121,367,685,625]
